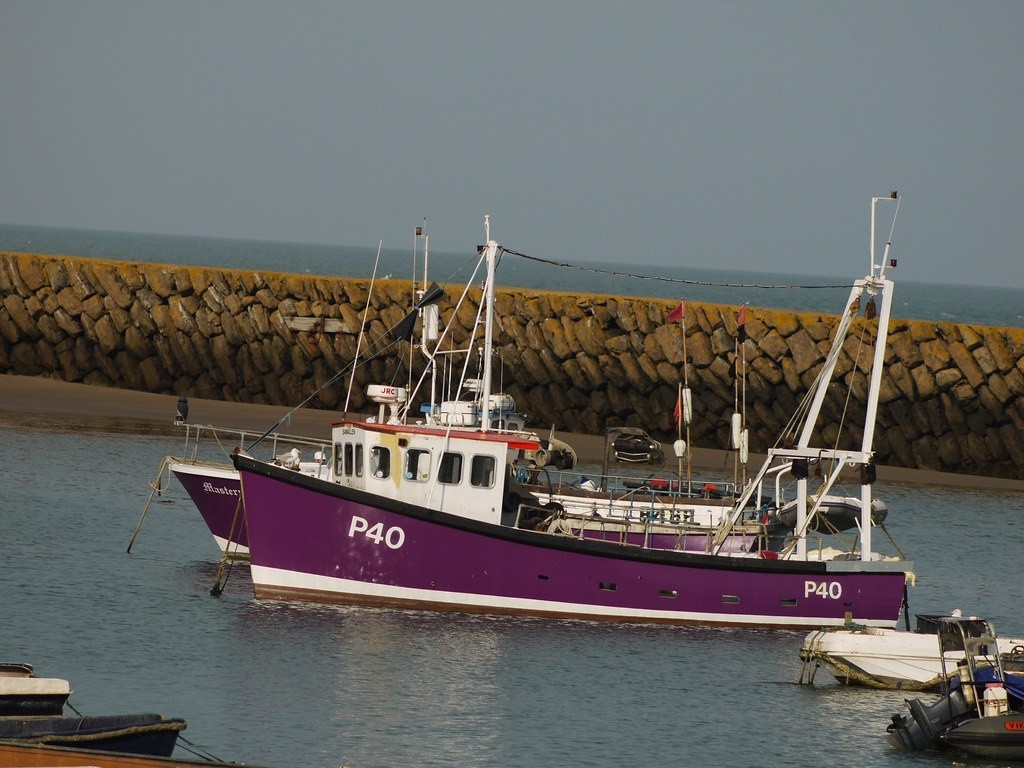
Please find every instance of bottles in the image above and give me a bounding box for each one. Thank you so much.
[983,683,1008,717]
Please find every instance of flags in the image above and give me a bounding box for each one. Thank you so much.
[738,305,745,325]
[674,397,679,432]
[737,325,746,344]
[667,303,682,323]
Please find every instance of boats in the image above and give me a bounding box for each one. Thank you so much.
[799,608,1024,692]
[0,661,273,768]
[126,188,915,631]
[881,615,1024,762]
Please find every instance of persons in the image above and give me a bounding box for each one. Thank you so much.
[315,451,326,464]
[703,483,721,499]
[651,477,668,486]
